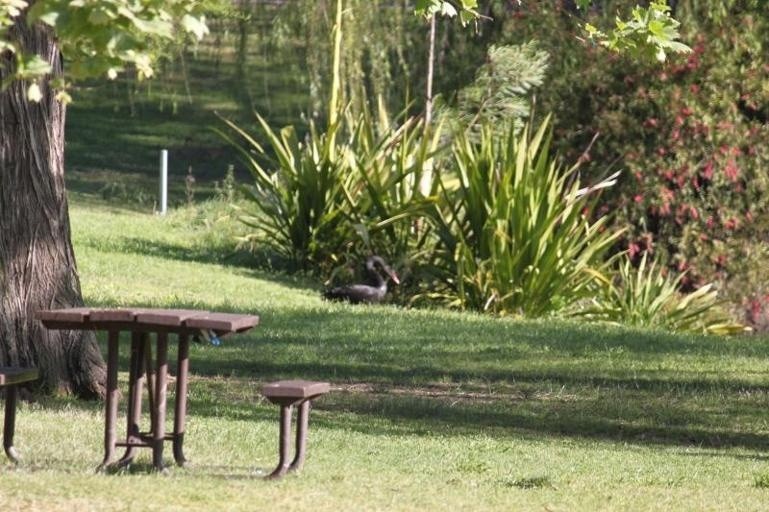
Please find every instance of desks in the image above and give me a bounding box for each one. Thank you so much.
[33,307,261,480]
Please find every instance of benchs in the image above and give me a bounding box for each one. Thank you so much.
[1,367,41,467]
[257,377,330,481]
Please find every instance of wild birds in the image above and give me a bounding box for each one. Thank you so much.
[323,253,400,306]
